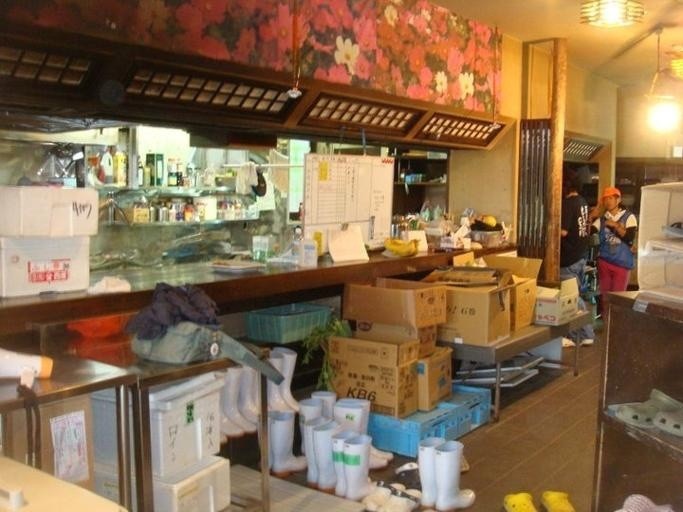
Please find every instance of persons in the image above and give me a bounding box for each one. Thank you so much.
[561,183,589,291]
[586,187,637,326]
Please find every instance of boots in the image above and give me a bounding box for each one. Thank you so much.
[418,436,446,508]
[339,398,393,461]
[334,405,387,470]
[226,366,263,426]
[273,346,302,413]
[222,366,257,433]
[345,434,378,500]
[215,370,244,436]
[362,483,405,511]
[435,441,476,512]
[304,415,332,488]
[394,461,421,490]
[311,391,337,421]
[266,358,296,413]
[379,489,422,512]
[332,429,349,497]
[259,413,273,473]
[300,398,322,453]
[270,411,307,478]
[313,421,344,494]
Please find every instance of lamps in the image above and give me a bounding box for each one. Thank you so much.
[644,29,681,133]
[579,0,644,32]
[665,43,683,82]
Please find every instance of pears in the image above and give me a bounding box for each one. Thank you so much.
[482,216,497,227]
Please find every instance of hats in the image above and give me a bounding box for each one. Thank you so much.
[601,187,620,199]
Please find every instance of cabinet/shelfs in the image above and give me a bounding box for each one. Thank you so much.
[0,327,139,511]
[592,291,683,512]
[637,181,683,305]
[26,304,270,511]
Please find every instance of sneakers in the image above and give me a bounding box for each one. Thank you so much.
[579,335,596,346]
[561,338,576,348]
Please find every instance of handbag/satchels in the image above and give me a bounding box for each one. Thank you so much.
[130,320,285,386]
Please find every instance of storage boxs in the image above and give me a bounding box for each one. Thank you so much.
[95,456,230,512]
[356,320,437,360]
[368,409,458,458]
[418,346,454,411]
[320,336,418,419]
[453,252,543,332]
[436,385,492,437]
[421,267,512,347]
[536,277,579,326]
[95,372,226,478]
[342,278,446,328]
[11,394,93,492]
[1,236,89,298]
[0,186,98,236]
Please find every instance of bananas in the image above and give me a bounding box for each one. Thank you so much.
[385,239,419,257]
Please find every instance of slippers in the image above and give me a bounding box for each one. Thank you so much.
[616,389,683,429]
[542,490,576,511]
[612,493,674,512]
[504,491,538,512]
[653,409,683,437]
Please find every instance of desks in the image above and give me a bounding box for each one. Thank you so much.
[439,309,596,422]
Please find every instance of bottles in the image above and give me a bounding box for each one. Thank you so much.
[292,227,304,258]
[168,163,178,186]
